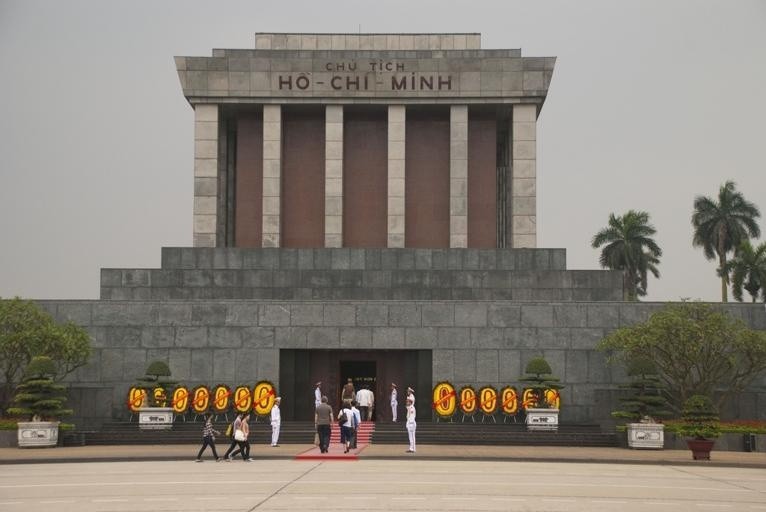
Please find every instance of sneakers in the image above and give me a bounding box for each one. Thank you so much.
[196,458,204,462]
[216,454,254,462]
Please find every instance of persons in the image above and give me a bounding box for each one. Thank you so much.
[269,397,281,448]
[407,386,416,405]
[390,382,398,422]
[224,410,251,462]
[336,378,375,454]
[227,411,252,462]
[405,398,417,453]
[314,396,335,453]
[314,381,323,410]
[195,413,224,463]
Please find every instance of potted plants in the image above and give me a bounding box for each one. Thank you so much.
[685,427,719,460]
[618,354,667,449]
[135,361,175,431]
[7,356,74,447]
[517,357,565,432]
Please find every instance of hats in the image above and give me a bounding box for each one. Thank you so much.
[407,387,415,401]
[274,397,282,402]
[316,381,322,385]
[392,382,396,387]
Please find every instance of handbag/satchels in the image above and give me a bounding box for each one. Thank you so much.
[224,423,233,438]
[235,429,248,442]
[339,408,347,427]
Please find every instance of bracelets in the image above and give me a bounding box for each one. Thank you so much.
[315,423,318,424]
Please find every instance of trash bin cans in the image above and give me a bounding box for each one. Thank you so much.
[744,432,756,452]
